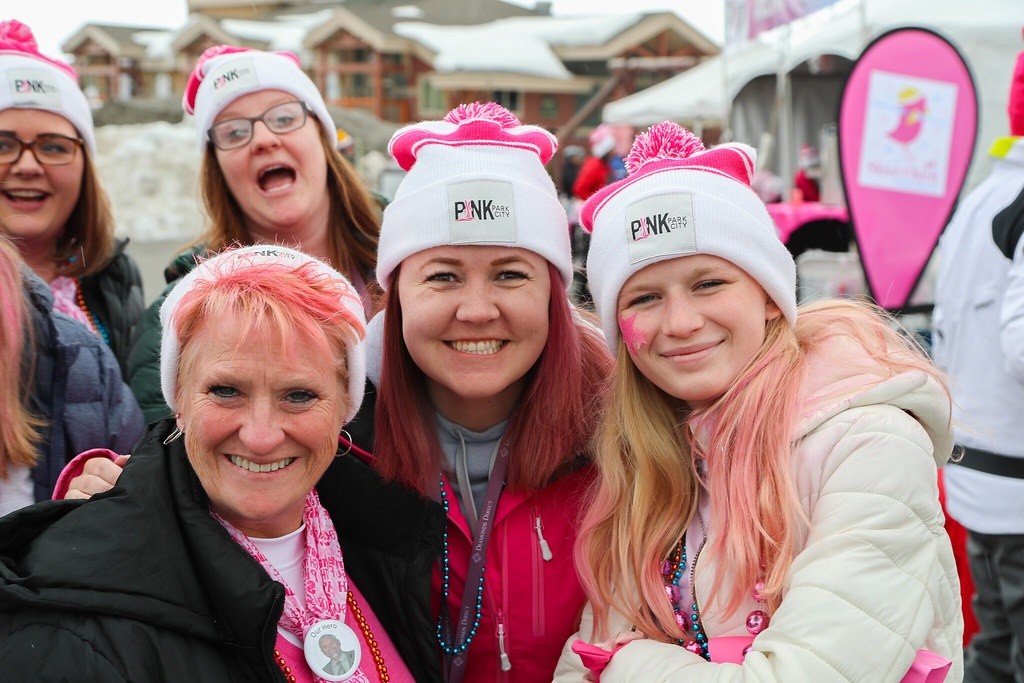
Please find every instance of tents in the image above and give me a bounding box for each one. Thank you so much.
[601,2,1024,329]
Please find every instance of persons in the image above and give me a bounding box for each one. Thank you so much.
[551,121,967,683]
[126,45,393,428]
[0,17,146,370]
[0,237,148,503]
[0,241,450,683]
[927,55,1024,683]
[50,101,626,682]
[318,634,355,675]
[794,143,821,201]
[561,125,641,304]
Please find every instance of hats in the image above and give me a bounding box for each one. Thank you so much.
[157,244,368,423]
[0,19,97,157]
[579,121,799,359]
[184,46,341,149]
[375,102,573,291]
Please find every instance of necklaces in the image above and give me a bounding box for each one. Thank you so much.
[275,588,391,683]
[65,256,110,343]
[659,515,713,659]
[434,471,505,655]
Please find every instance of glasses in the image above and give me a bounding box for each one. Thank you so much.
[206,99,304,152]
[0,133,84,167]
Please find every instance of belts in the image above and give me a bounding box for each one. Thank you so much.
[947,444,1024,479]
